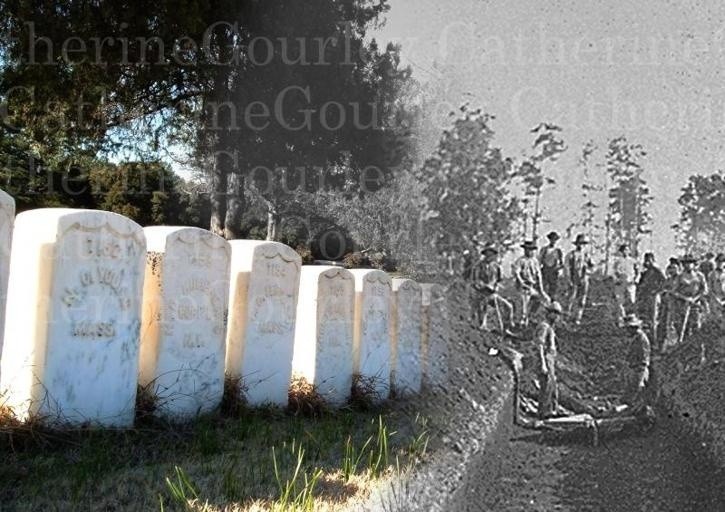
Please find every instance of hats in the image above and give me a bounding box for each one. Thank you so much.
[678,254,699,263]
[622,314,642,327]
[481,231,589,256]
[544,302,566,315]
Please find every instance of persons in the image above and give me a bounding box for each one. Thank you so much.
[467,230,725,422]
